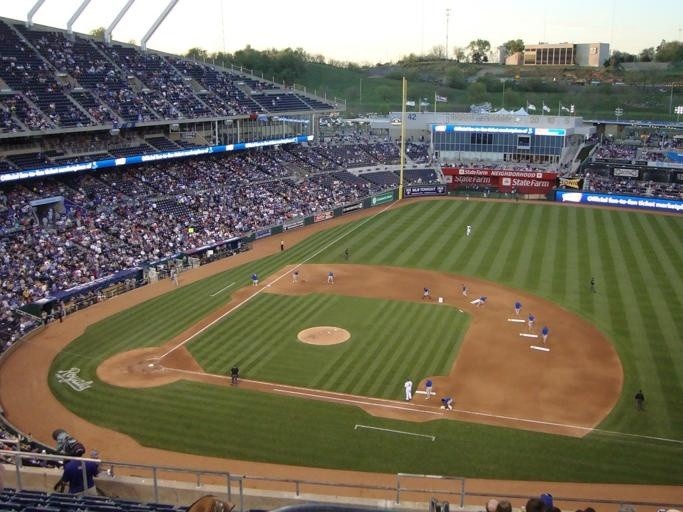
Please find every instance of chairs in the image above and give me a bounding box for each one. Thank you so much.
[1,19,346,141]
[1,417,382,511]
[1,133,245,362]
[579,138,682,203]
[237,106,450,242]
